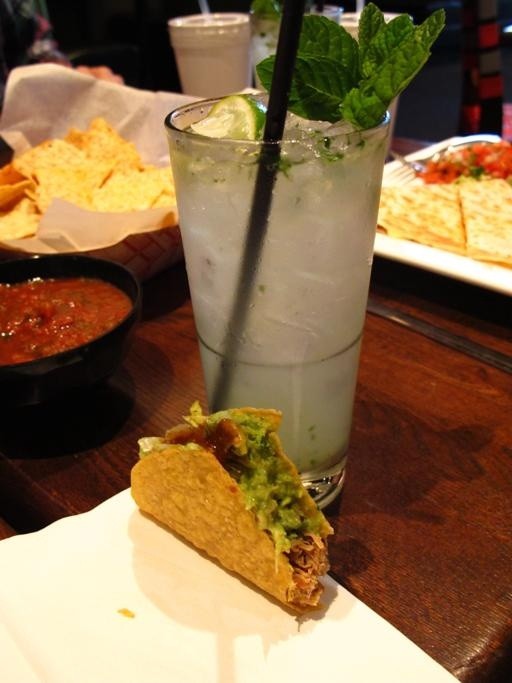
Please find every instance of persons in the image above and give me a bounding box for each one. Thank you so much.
[0,0,125,167]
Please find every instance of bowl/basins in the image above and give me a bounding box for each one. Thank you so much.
[0,255,143,417]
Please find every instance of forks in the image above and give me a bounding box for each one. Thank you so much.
[391,137,502,178]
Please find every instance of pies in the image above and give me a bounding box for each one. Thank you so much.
[131,408,334,614]
[378,170,512,264]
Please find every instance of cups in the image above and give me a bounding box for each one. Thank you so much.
[167,12,254,97]
[311,4,344,25]
[164,86,391,513]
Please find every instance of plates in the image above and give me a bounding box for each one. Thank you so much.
[374,130,512,300]
[1,483,462,683]
[1,223,182,283]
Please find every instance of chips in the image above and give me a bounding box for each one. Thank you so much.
[0,117,175,239]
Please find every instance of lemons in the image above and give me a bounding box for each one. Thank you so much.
[207,94,265,140]
